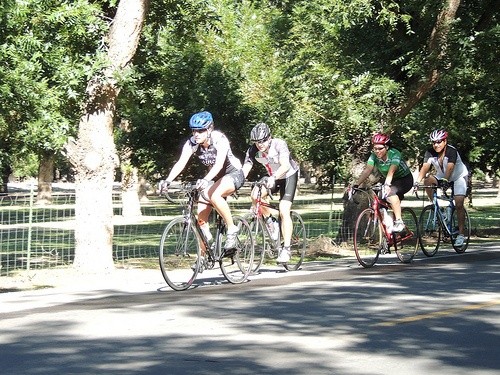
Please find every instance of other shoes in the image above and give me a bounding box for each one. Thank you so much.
[375,241,388,251]
[392,220,406,233]
[268,220,280,241]
[191,255,208,270]
[432,214,447,225]
[224,225,239,250]
[454,234,465,247]
[275,249,292,263]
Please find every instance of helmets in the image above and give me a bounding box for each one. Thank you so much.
[250,123,271,142]
[430,129,448,142]
[189,111,213,130]
[372,133,390,146]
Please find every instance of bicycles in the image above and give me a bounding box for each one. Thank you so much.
[351,183,420,268]
[413,181,470,256]
[156,185,254,291]
[230,181,306,276]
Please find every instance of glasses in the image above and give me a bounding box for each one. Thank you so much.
[373,146,386,150]
[432,140,443,143]
[191,126,210,133]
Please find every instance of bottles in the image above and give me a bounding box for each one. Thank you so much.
[380,208,394,233]
[440,206,452,223]
[266,217,279,241]
[198,219,213,243]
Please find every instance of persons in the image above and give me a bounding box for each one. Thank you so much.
[347,134,414,246]
[158,111,245,270]
[243,123,299,262]
[413,129,471,246]
